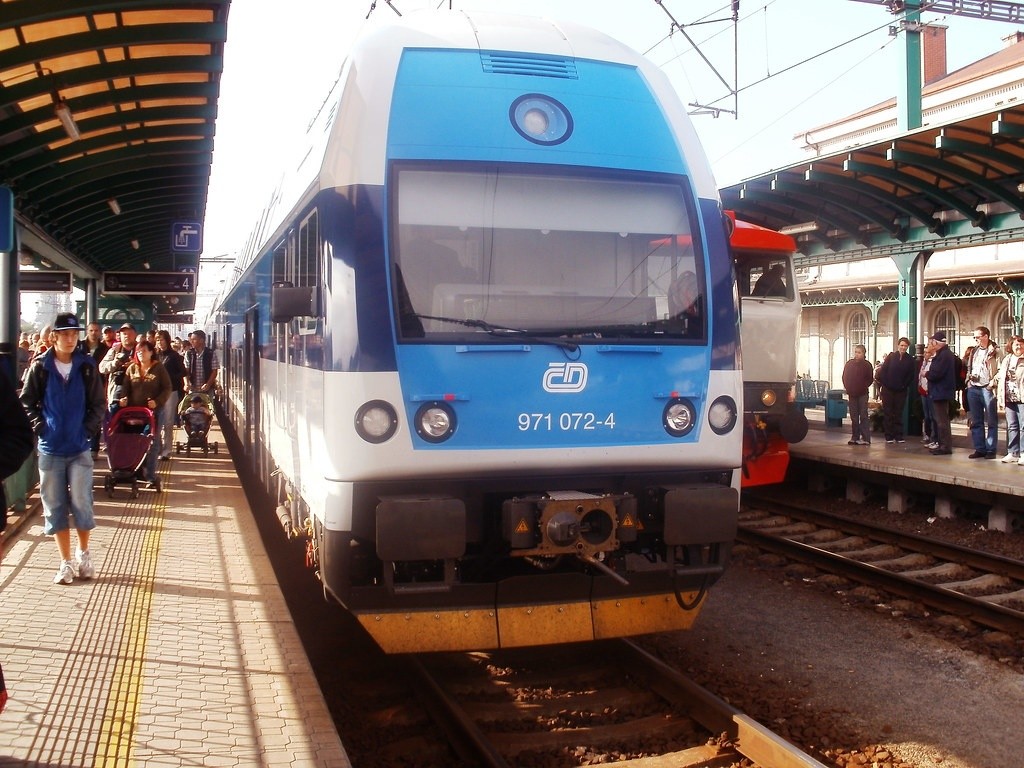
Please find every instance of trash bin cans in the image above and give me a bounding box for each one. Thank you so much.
[824,389,848,427]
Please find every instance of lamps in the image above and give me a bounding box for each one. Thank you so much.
[56,103,80,138]
[108,199,121,214]
[144,263,150,269]
[131,240,139,250]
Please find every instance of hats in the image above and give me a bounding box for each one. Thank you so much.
[930,332,946,342]
[117,323,136,331]
[52,312,85,331]
[102,326,114,334]
[190,396,202,403]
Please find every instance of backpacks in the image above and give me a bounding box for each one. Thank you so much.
[938,348,966,391]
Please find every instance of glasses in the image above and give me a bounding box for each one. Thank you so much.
[136,349,148,353]
[973,336,982,340]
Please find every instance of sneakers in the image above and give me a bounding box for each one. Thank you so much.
[54,559,75,583]
[75,547,95,580]
[1017,454,1024,466]
[1002,454,1019,462]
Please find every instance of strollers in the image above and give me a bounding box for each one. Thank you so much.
[175,391,219,457]
[104,396,164,500]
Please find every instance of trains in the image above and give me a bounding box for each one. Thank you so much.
[648,208,811,490]
[203,9,743,662]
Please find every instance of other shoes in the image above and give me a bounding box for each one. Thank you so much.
[886,440,894,444]
[921,435,930,443]
[984,453,996,459]
[929,449,952,455]
[925,442,939,449]
[90,451,98,460]
[191,431,196,437]
[848,440,870,445]
[896,440,906,443]
[969,451,985,458]
[157,454,169,460]
[199,431,204,437]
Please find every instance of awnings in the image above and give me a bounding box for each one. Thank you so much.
[0,0,232,342]
[718,98,1024,355]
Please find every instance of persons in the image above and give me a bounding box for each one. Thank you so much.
[19,311,108,583]
[965,326,1004,459]
[110,340,172,489]
[842,345,873,444]
[397,223,468,330]
[0,355,34,561]
[18,321,221,460]
[880,338,914,443]
[750,264,791,296]
[918,331,975,455]
[997,335,1024,465]
[872,353,887,402]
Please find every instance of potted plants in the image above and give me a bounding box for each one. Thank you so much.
[868,389,961,437]
